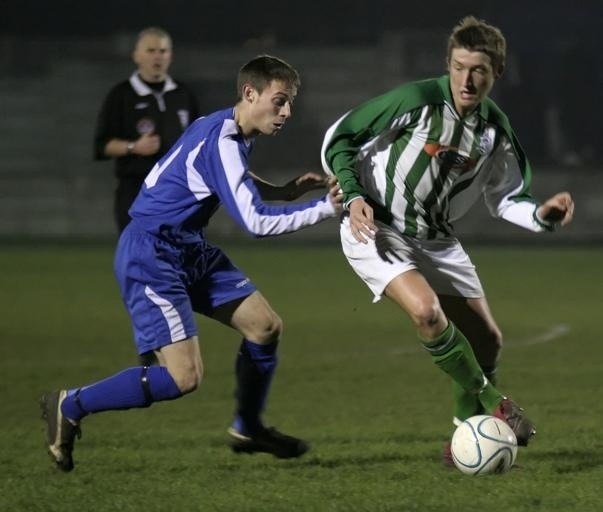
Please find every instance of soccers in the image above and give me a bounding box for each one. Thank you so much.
[450,415,517,476]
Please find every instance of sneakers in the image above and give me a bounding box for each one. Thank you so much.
[225,423,309,461]
[491,393,538,448]
[36,387,84,475]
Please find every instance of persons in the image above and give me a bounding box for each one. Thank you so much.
[36,52,343,472]
[320,14,577,463]
[91,24,198,228]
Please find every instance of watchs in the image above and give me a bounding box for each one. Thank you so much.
[127,140,135,154]
